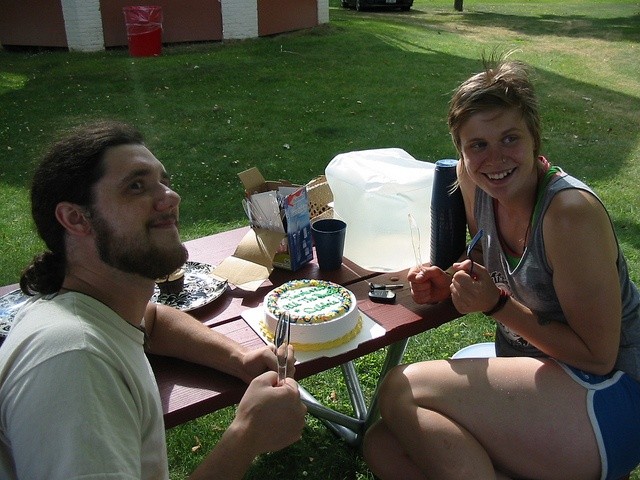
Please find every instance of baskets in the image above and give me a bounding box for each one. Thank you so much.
[305,175,334,247]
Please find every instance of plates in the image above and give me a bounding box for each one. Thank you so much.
[0,287,39,337]
[148,261,229,312]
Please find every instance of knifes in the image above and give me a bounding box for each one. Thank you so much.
[275,311,289,386]
[407,212,423,270]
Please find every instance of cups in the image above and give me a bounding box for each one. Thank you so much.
[311,219,346,270]
[431,159,467,271]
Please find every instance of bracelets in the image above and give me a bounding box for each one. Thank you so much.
[482,287,509,316]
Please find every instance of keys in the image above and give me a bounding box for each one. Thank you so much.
[367,281,405,303]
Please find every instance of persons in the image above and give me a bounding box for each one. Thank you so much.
[360,56,639,479]
[0,122,309,478]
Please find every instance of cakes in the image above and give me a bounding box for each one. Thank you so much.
[258,279,362,353]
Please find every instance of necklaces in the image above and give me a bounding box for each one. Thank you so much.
[62,286,153,351]
[493,161,541,256]
